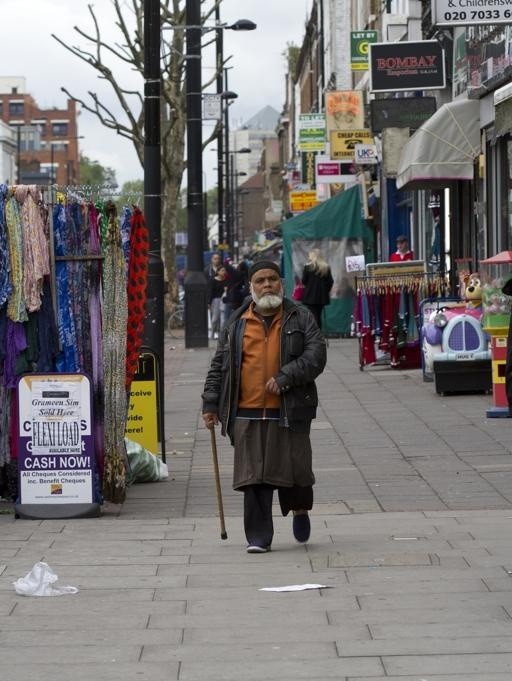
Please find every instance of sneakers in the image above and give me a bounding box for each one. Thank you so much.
[292,509,312,542]
[245,541,271,553]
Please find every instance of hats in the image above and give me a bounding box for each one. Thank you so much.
[248,261,281,277]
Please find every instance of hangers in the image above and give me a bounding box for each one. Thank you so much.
[6,185,144,214]
[354,273,451,297]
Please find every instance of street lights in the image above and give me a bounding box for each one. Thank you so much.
[204,88,238,101]
[139,17,259,442]
[223,171,256,176]
[216,144,252,242]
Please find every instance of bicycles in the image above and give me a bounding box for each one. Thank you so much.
[167,302,185,341]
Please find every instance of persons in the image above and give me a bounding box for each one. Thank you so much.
[201,261,327,554]
[389,236,413,262]
[205,265,229,340]
[303,249,333,334]
[223,262,250,326]
[204,254,222,332]
